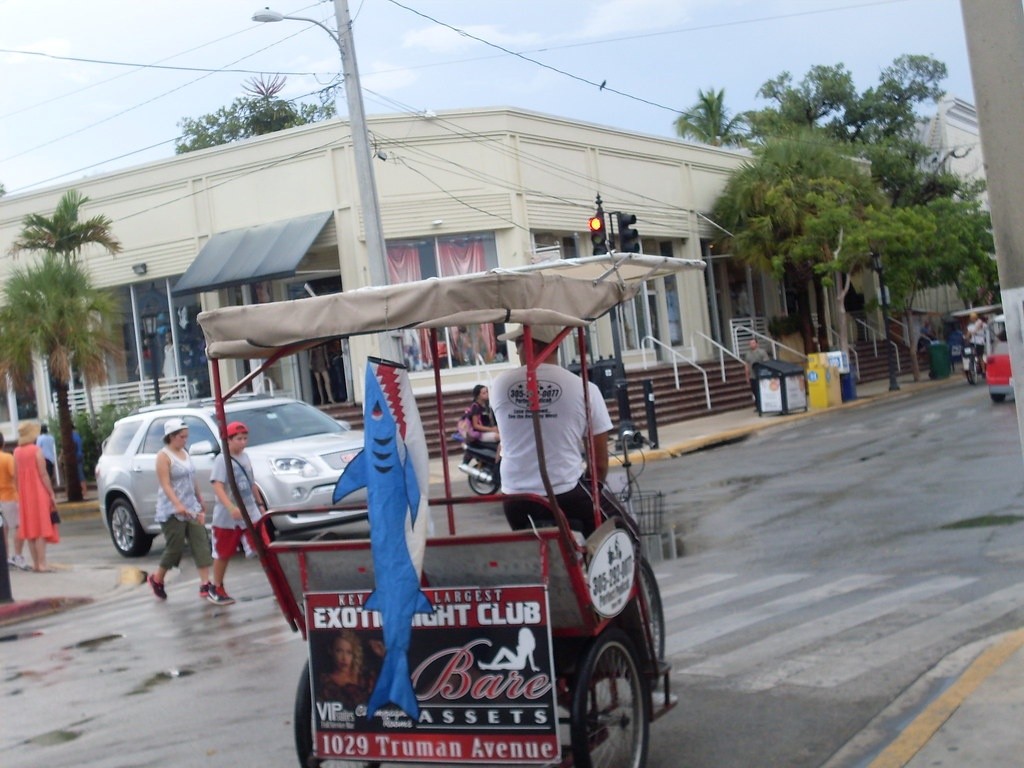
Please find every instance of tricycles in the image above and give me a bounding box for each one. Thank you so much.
[191,251,705,768]
[985,314,1015,404]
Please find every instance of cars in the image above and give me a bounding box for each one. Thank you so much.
[93,392,368,558]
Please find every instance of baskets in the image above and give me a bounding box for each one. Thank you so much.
[613,489,665,535]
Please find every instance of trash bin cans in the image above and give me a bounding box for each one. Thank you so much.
[753,360,808,416]
[927,343,951,379]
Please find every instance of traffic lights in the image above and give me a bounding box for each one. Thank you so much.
[616,213,642,254]
[587,217,607,257]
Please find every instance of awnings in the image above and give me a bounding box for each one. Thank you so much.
[172,211,338,296]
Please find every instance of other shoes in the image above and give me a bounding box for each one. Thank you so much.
[9,553,28,569]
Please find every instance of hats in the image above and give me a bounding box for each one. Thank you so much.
[17,421,41,445]
[226,422,248,436]
[497,322,561,347]
[162,418,188,442]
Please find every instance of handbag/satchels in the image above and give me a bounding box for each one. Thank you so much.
[51,509,60,523]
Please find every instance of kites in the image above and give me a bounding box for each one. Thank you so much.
[332,356,432,723]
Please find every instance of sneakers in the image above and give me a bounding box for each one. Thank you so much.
[200,582,213,596]
[206,585,236,605]
[148,574,167,600]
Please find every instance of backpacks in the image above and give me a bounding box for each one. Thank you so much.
[457,403,484,443]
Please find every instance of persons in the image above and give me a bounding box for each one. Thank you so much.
[918,320,935,350]
[745,338,768,412]
[965,313,986,377]
[72,425,88,500]
[146,418,214,600]
[208,422,271,606]
[13,423,60,572]
[36,426,54,478]
[484,322,678,711]
[0,433,31,570]
[469,385,502,465]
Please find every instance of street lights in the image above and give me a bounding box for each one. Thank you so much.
[246,10,403,375]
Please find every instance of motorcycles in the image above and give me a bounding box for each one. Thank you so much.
[450,431,505,495]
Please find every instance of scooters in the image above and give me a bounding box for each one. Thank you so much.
[959,337,986,386]
